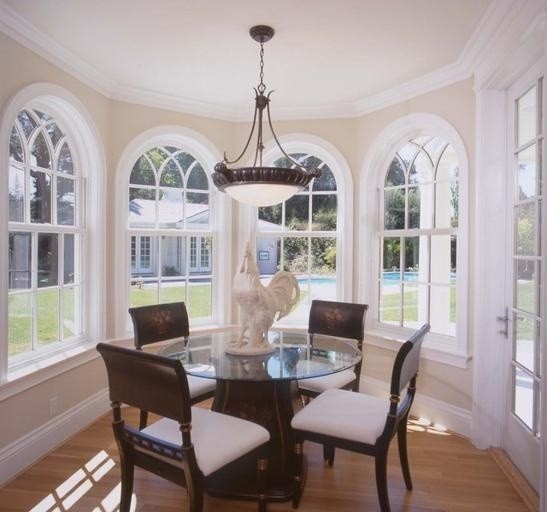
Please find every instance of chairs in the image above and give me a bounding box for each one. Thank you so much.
[95,342,270,512]
[289,323,431,511]
[128,301,216,431]
[294,300,369,406]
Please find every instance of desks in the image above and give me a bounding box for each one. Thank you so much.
[157,331,363,503]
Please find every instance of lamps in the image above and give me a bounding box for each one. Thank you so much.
[211,25,321,208]
